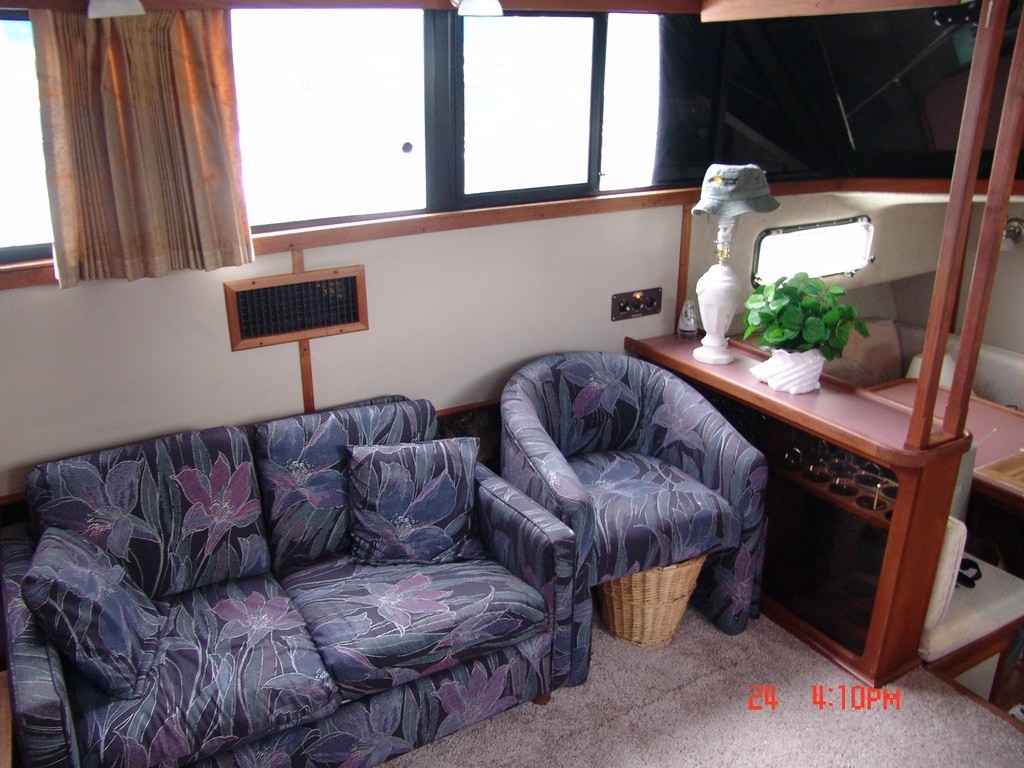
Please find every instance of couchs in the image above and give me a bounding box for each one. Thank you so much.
[500,351,767,688]
[0,396,577,768]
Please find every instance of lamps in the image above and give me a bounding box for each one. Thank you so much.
[693,211,741,364]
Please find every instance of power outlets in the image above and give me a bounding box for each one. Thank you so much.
[612,287,662,322]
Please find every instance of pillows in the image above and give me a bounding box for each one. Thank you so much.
[19,524,169,702]
[253,399,437,571]
[24,423,272,596]
[342,437,477,564]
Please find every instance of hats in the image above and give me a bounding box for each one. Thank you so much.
[691,163,780,218]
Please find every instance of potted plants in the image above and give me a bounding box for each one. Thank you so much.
[742,272,869,396]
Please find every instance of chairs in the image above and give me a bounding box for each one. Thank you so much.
[920,515,1024,702]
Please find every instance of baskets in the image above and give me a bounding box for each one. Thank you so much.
[598,553,708,646]
